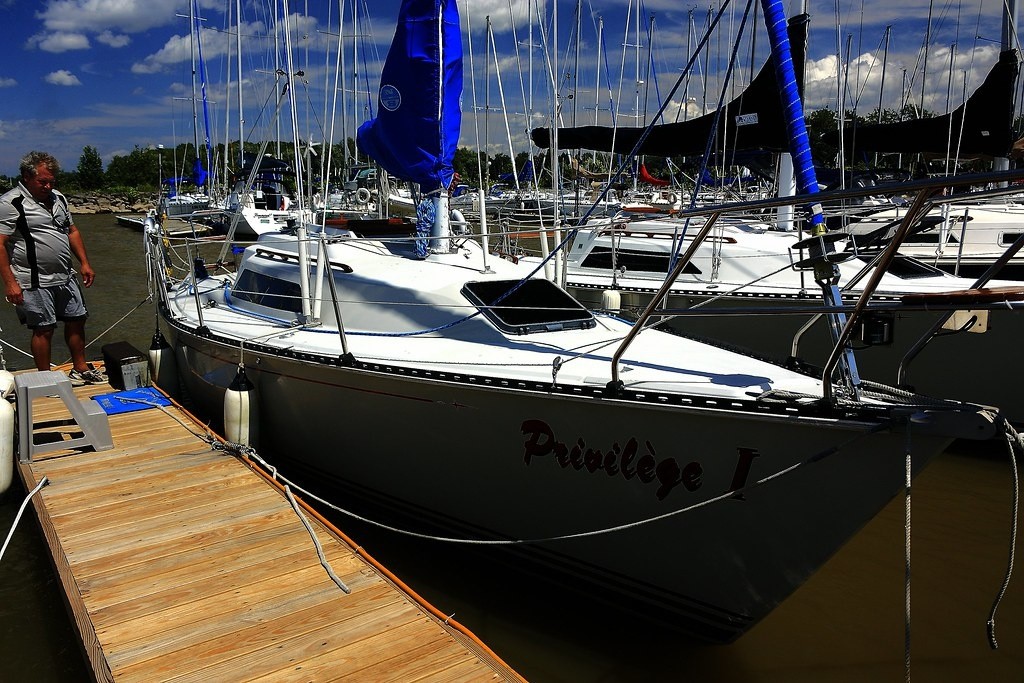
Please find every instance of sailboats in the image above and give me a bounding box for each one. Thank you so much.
[138,0,1024,645]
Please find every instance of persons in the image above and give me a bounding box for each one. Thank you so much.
[1,150,109,386]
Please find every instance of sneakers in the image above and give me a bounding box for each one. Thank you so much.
[68,368,109,386]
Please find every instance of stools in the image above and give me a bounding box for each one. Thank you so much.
[14,371,116,465]
